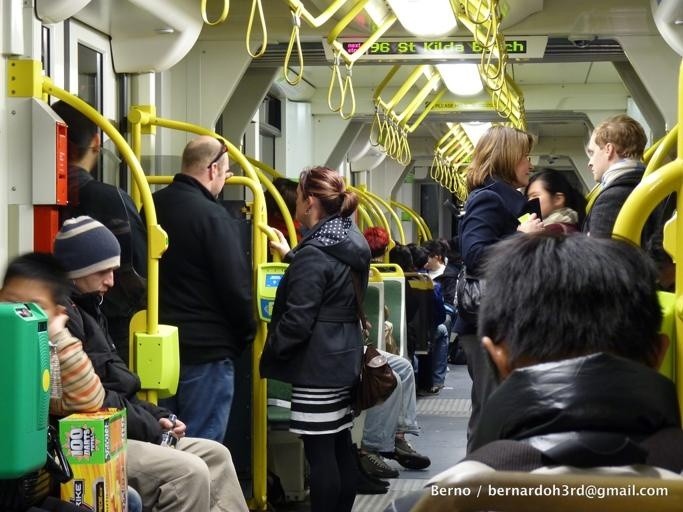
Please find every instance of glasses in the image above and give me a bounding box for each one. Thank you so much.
[206,137,229,169]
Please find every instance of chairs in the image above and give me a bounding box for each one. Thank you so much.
[265,261,436,503]
[410,469,683,511]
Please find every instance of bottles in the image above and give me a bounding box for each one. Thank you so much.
[48,345,62,400]
[156,413,178,449]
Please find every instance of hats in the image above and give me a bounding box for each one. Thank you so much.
[54,214,124,282]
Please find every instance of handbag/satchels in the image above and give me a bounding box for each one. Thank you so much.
[447,335,468,365]
[456,263,482,319]
[351,344,398,411]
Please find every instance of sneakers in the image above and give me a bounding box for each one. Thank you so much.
[416,386,441,396]
[357,430,432,479]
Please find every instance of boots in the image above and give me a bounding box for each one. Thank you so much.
[347,441,391,496]
